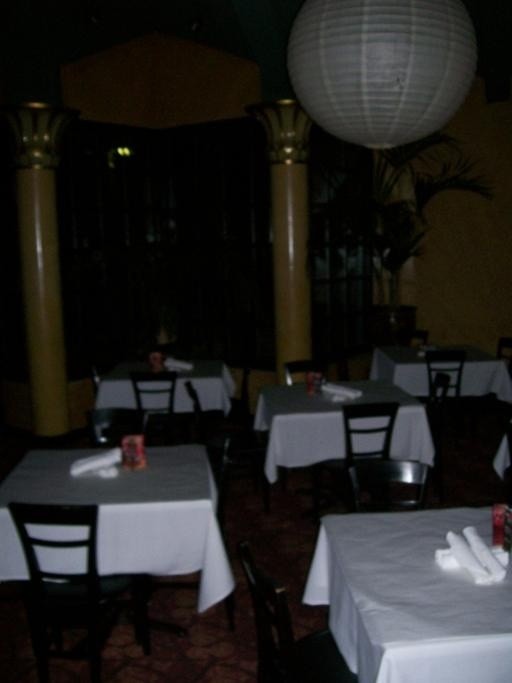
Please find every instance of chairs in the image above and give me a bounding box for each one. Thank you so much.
[8,329,511,683]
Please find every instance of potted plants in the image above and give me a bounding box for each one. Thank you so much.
[318,131,494,348]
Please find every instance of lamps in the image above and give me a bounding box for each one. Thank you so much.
[289,0,478,149]
